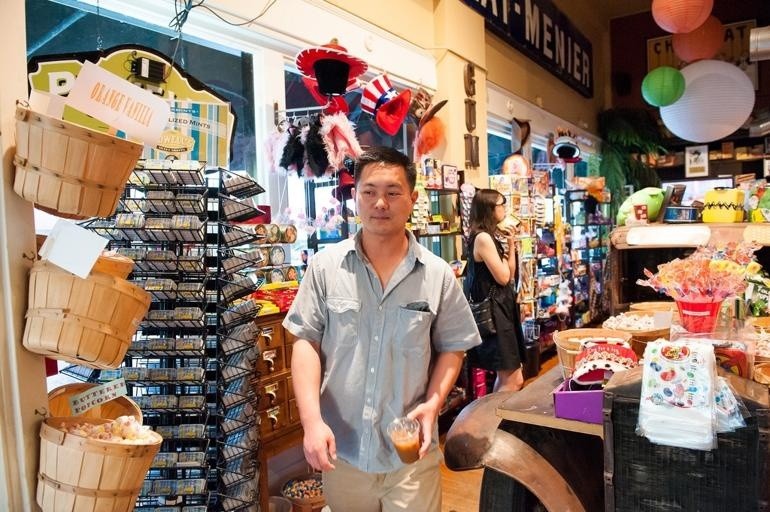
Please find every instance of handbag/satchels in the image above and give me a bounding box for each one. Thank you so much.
[469,299,495,333]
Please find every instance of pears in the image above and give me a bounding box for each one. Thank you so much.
[58,416,138,443]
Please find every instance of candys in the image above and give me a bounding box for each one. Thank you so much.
[283,478,323,499]
[634,241,761,302]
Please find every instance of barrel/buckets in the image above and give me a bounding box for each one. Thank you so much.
[21,260,152,370]
[47,382,144,426]
[34,233,136,280]
[552,327,632,381]
[628,300,677,311]
[602,311,671,359]
[33,414,164,512]
[11,104,146,218]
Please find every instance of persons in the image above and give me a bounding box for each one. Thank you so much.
[460,189,525,395]
[281,149,483,512]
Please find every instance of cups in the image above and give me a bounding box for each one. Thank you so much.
[386,416,422,465]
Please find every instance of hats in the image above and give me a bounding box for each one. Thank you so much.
[552,135,580,158]
[296,38,448,136]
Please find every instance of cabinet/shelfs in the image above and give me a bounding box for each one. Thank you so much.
[251,311,303,512]
[60,157,266,512]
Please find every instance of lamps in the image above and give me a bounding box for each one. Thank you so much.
[641,1,756,147]
